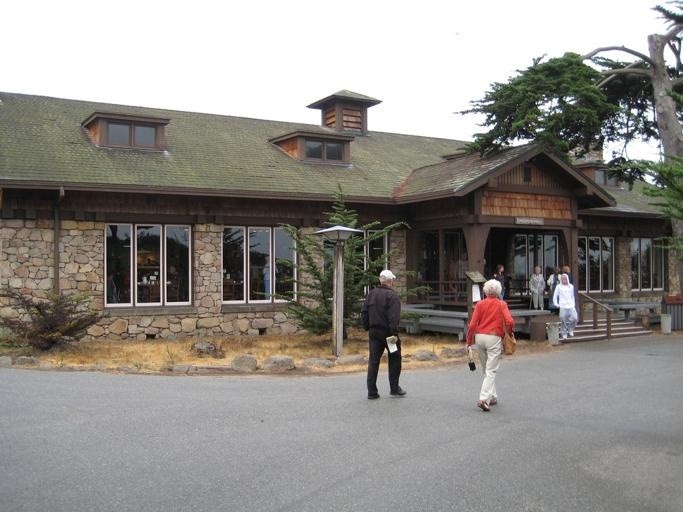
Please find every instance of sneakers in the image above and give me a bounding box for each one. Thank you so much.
[391,388,406,395]
[368,393,380,399]
[563,331,574,340]
[478,399,496,412]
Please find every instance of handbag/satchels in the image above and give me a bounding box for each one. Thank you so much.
[504,332,516,355]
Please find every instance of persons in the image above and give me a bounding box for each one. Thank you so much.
[529,266,546,311]
[448,253,468,301]
[494,264,505,299]
[547,265,577,340]
[465,278,514,411]
[361,269,407,398]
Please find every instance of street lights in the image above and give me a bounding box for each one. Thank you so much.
[313,226,363,357]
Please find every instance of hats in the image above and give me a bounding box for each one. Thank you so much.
[380,270,396,280]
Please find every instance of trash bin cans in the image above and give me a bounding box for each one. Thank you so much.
[660,295,683,330]
